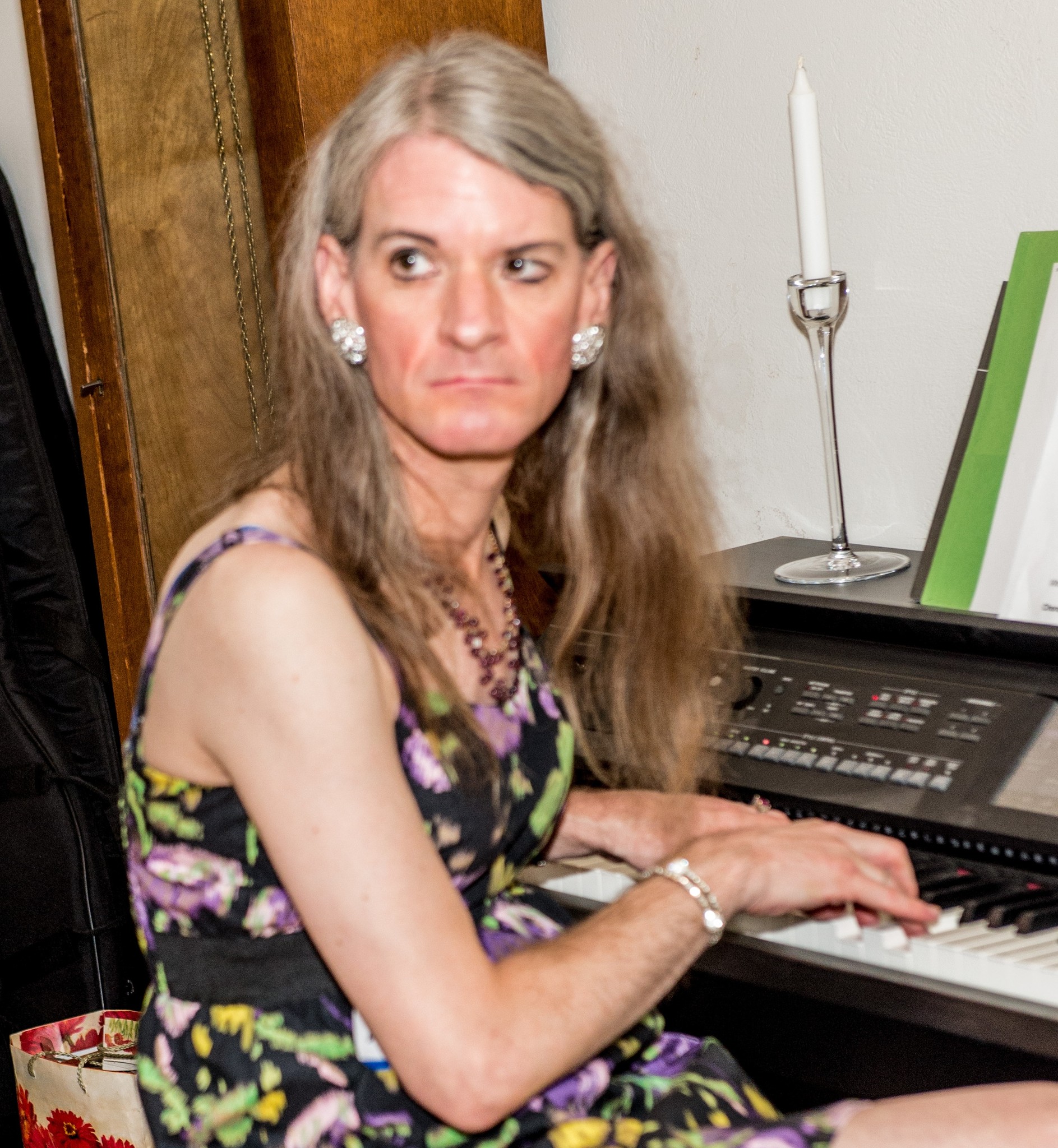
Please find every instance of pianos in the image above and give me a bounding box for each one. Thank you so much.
[527,536,1058,1065]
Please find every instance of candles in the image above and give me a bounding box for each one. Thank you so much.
[787,50,833,311]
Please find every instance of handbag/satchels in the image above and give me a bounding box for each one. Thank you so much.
[8,1007,155,1148]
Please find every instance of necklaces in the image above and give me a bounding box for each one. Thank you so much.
[424,517,521,701]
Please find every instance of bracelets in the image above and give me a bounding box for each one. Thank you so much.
[632,856,726,944]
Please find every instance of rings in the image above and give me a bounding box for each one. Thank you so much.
[751,794,772,818]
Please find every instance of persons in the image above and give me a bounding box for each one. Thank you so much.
[115,29,1058,1148]
